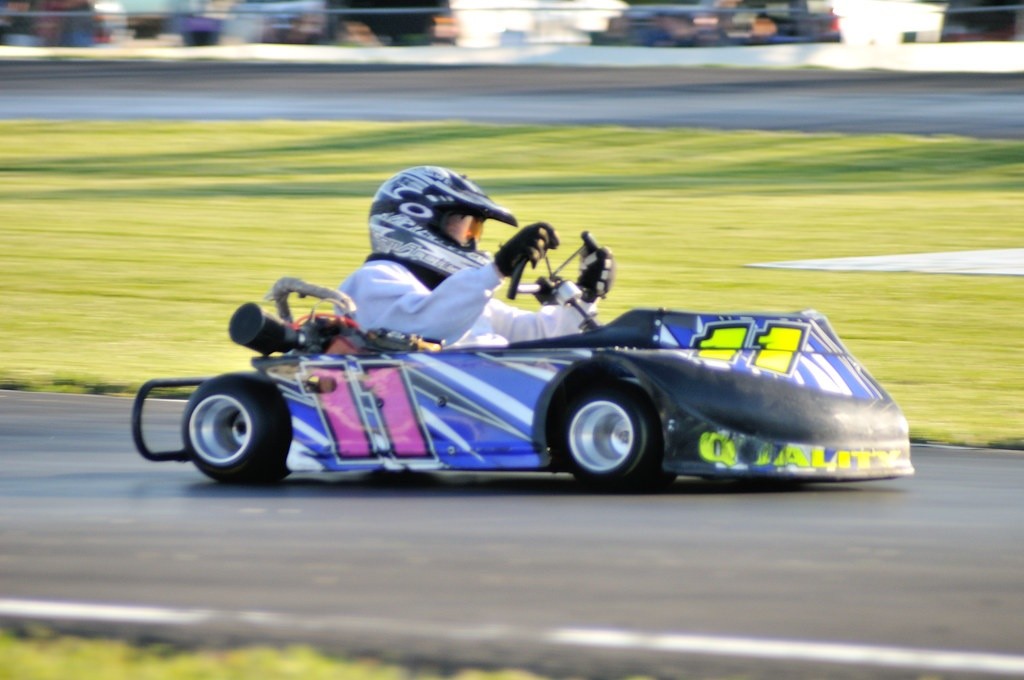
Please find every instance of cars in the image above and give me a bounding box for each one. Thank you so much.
[588,2,842,46]
[940,1,1024,43]
[230,1,461,46]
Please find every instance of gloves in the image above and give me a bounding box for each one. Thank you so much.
[575,231,615,303]
[493,221,560,277]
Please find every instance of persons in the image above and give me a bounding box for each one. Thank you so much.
[334,166,613,351]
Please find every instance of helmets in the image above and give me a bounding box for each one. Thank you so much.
[369,165,518,276]
